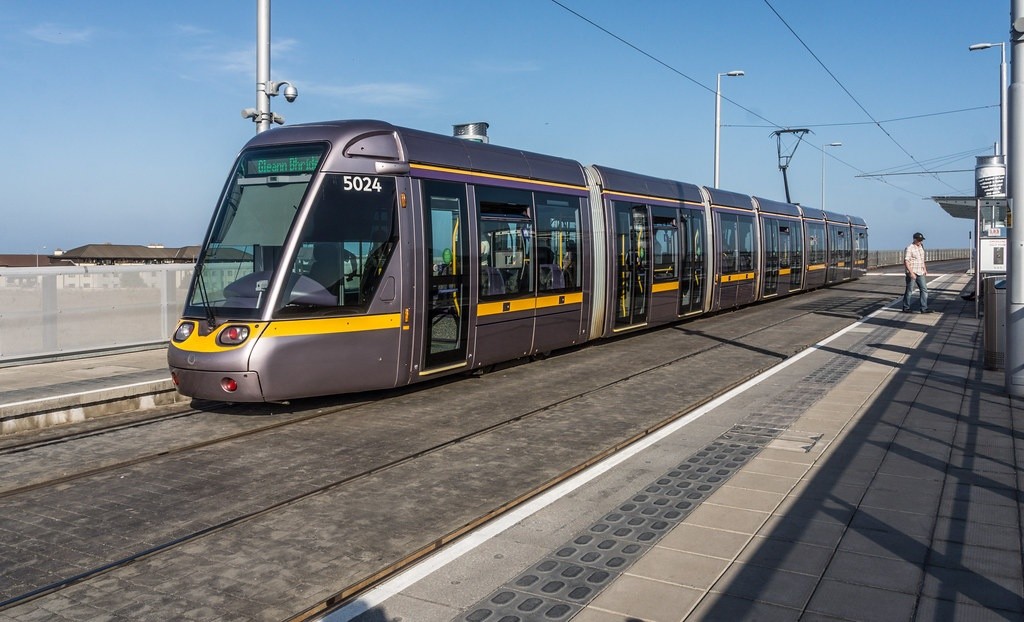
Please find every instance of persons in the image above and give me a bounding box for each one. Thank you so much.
[960,291,975,302]
[902,233,933,314]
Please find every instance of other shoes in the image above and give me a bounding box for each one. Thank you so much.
[921,309,934,313]
[903,310,913,313]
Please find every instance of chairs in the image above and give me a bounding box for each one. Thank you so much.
[309,243,339,294]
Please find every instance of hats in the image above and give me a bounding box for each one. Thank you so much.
[914,233,925,240]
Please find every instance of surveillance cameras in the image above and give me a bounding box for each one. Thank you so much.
[284,87,297,104]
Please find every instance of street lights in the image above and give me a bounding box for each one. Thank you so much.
[821,143,842,210]
[969,42,1008,166]
[713,71,745,189]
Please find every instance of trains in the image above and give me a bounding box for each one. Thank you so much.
[165,117,869,407]
